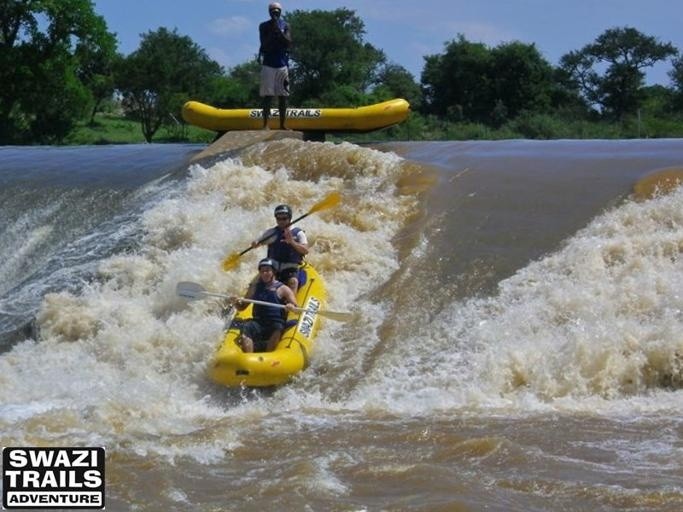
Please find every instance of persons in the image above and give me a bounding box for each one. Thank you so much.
[228,204,310,353]
[258,2,293,130]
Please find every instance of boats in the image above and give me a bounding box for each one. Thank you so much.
[207,259,327,388]
[181,98,412,130]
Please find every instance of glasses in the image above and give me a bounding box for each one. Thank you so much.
[276,216,289,220]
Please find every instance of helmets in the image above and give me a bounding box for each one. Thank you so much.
[258,257,279,272]
[268,2,281,12]
[275,204,292,217]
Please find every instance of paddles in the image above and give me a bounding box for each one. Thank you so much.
[224,192,341,272]
[175,282,351,321]
